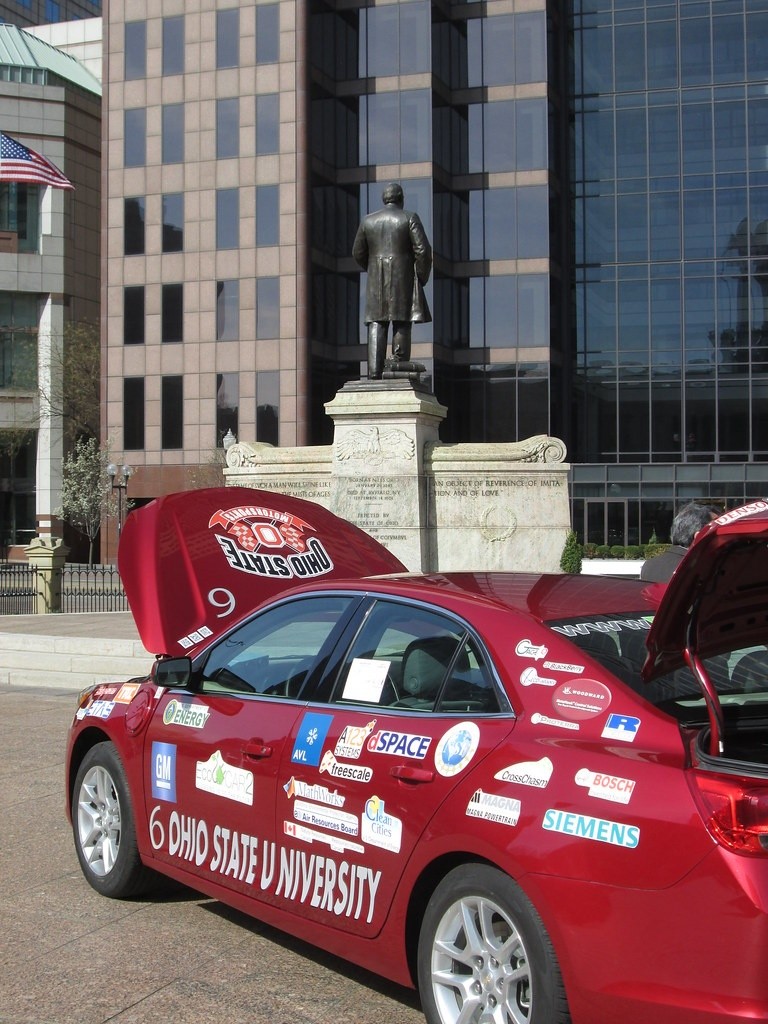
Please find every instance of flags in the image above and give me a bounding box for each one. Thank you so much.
[0,132,76,191]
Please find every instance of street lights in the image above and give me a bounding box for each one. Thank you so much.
[107,463,132,540]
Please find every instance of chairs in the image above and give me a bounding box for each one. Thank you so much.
[389,635,472,714]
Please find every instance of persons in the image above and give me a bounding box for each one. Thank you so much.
[641,502,736,698]
[352,182,432,381]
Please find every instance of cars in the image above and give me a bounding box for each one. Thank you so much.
[62,486,768,1024]
